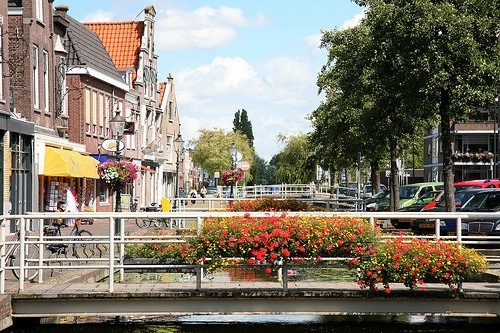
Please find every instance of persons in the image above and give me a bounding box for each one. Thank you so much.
[200,186,208,203]
[189,188,197,206]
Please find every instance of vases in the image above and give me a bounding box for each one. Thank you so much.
[123,258,195,273]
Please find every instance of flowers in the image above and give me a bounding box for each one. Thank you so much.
[122,213,488,294]
[219,169,244,186]
[94,161,138,192]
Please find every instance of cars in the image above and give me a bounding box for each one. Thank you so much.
[302,179,500,250]
[206,185,242,196]
[179,188,188,205]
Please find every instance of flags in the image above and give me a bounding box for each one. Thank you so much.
[146,141,154,149]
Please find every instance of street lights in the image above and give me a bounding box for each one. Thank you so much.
[108,106,127,239]
[228,141,239,203]
[172,131,186,207]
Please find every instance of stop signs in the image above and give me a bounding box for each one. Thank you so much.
[240,161,250,171]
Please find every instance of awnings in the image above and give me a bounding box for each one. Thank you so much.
[43,147,101,179]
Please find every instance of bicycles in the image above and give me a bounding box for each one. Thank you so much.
[0,220,40,282]
[130,196,169,229]
[36,216,96,259]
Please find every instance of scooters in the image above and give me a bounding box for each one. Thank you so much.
[190,192,197,204]
[200,193,207,203]
